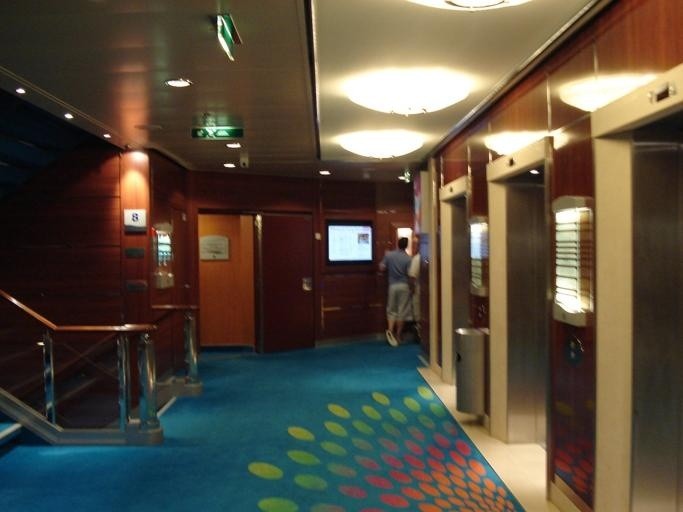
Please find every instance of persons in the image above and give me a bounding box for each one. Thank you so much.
[407,236,424,345]
[379,238,412,347]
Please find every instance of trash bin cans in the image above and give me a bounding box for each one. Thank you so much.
[454,327,486,416]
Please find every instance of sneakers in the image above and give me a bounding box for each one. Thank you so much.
[386,330,398,347]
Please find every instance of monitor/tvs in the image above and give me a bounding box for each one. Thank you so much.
[324,219,375,266]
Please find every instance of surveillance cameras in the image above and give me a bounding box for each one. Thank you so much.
[239,156,250,169]
